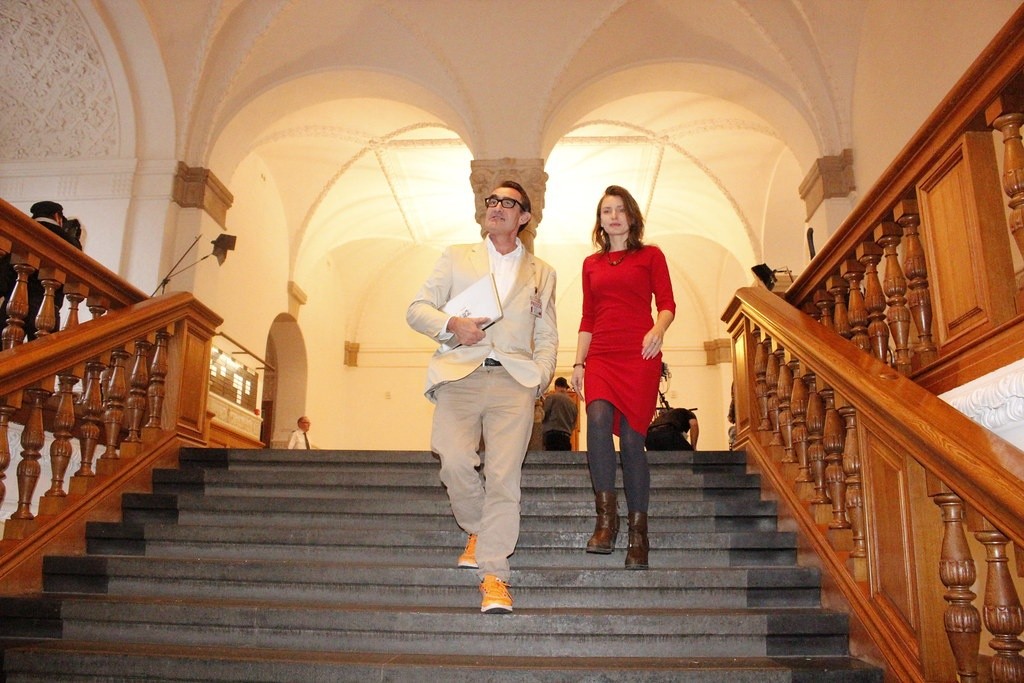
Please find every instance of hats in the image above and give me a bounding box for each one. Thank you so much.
[30,201,68,225]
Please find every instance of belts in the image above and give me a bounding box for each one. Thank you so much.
[481,358,502,366]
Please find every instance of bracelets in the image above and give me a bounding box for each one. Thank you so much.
[573,363,585,369]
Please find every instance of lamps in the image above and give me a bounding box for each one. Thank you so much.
[750,263,793,292]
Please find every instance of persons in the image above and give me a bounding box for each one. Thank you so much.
[570,185,677,571]
[644,406,699,451]
[539,376,579,451]
[404,179,559,617]
[727,381,737,441]
[0,199,81,358]
[287,416,313,450]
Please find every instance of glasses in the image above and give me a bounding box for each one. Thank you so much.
[485,198,526,212]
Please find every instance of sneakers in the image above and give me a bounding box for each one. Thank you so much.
[479,575,514,614]
[457,533,479,569]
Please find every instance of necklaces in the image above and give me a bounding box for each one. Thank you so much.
[605,246,629,265]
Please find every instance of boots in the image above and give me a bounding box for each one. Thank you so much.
[624,511,650,570]
[586,489,621,553]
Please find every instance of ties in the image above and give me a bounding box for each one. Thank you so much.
[303,433,310,449]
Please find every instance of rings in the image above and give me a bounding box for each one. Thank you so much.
[651,342,657,345]
